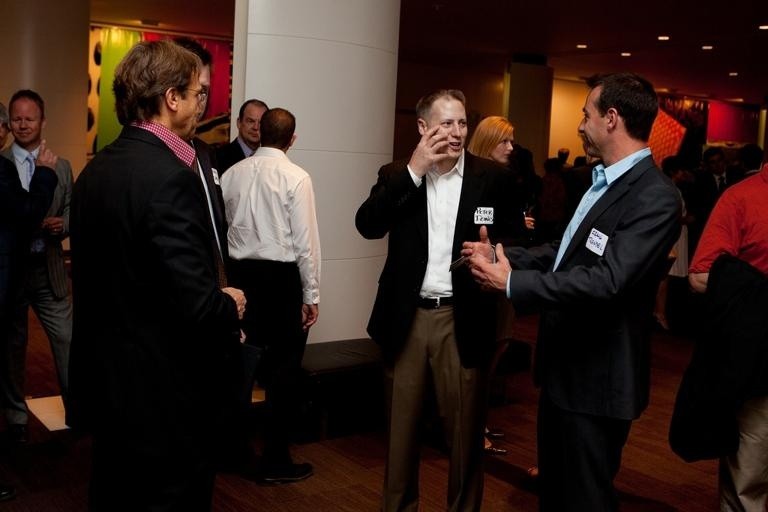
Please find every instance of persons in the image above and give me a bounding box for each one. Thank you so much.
[461,71,685,509]
[65,40,248,512]
[173,37,250,345]
[686,122,767,511]
[355,86,510,510]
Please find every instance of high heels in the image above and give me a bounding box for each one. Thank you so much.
[488,424,507,440]
[485,439,508,459]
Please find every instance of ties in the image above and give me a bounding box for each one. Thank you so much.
[23,155,44,254]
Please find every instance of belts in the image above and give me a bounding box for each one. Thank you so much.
[416,294,454,311]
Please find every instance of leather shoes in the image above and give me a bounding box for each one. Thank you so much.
[254,460,316,487]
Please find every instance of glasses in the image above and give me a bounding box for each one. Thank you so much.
[177,87,208,104]
